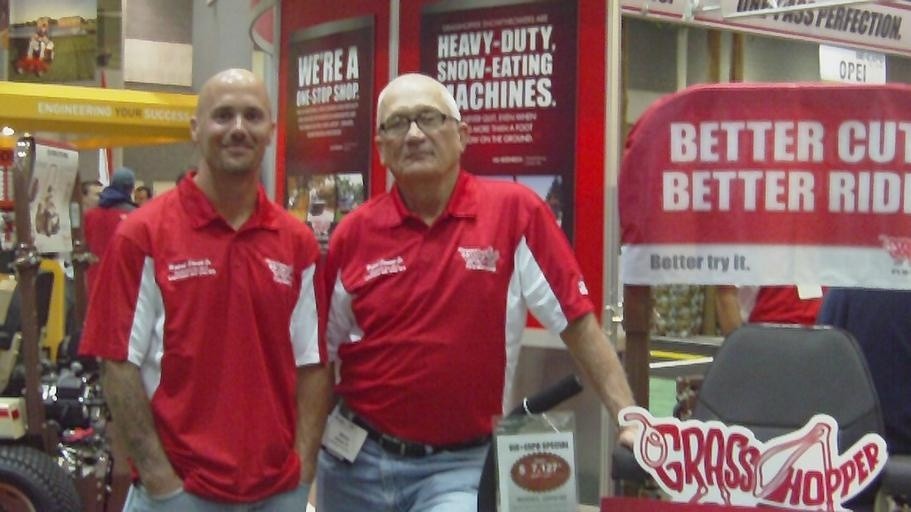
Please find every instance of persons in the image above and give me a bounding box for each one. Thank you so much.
[717,285,829,337]
[313,73,643,512]
[816,287,910,460]
[76,68,333,512]
[79,167,154,304]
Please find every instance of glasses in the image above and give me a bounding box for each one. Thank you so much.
[374,108,460,142]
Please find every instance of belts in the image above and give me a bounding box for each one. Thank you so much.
[336,398,491,457]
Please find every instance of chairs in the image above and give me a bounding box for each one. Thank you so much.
[611,324,910,512]
